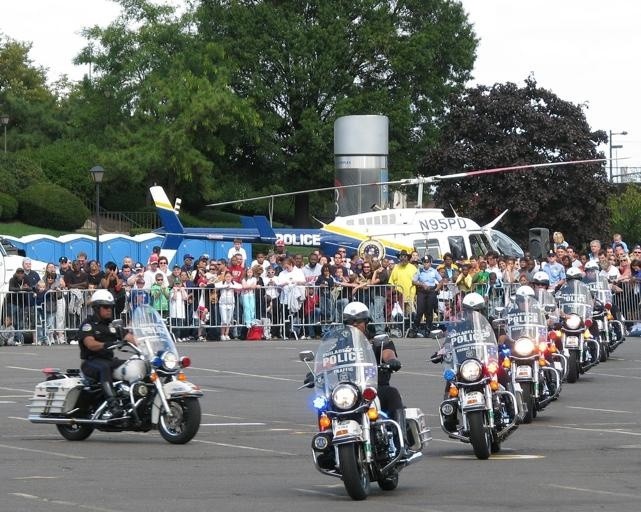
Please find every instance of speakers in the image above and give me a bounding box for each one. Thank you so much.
[528,227,550,259]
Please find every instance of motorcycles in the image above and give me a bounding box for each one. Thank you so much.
[499,304,559,419]
[429,325,524,459]
[27,302,207,445]
[300,319,412,497]
[545,304,602,381]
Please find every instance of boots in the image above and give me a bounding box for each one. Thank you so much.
[392,410,412,458]
[318,416,337,471]
[103,381,126,418]
[504,382,521,425]
[444,392,457,432]
[613,322,625,341]
[592,337,599,363]
[545,363,557,396]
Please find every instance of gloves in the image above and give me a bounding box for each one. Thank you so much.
[304,374,315,388]
[386,359,401,372]
[104,341,117,351]
[431,352,443,364]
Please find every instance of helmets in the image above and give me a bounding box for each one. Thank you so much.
[516,286,535,297]
[533,271,549,282]
[584,260,600,270]
[566,267,583,278]
[343,301,370,321]
[462,292,485,310]
[91,289,116,307]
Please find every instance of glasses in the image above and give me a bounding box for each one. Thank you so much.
[268,272,274,275]
[100,305,113,308]
[346,319,363,325]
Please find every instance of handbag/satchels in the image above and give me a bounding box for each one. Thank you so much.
[246,317,263,340]
[261,318,272,340]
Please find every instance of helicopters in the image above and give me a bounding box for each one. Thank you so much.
[145,159,632,296]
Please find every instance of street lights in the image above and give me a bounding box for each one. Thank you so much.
[88,163,108,262]
[607,127,630,181]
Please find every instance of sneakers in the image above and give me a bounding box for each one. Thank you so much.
[177,335,313,342]
[15,340,80,346]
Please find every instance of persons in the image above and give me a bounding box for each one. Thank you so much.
[532,261,623,381]
[502,285,558,400]
[436,292,513,431]
[1,230,641,345]
[304,299,409,469]
[80,290,141,415]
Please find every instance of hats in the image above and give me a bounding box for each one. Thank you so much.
[135,254,208,284]
[396,250,412,261]
[59,256,68,262]
[275,240,285,246]
[422,256,431,262]
[16,268,24,273]
[547,250,556,256]
[355,259,364,265]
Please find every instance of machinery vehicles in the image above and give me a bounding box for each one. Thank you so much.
[577,302,625,369]
